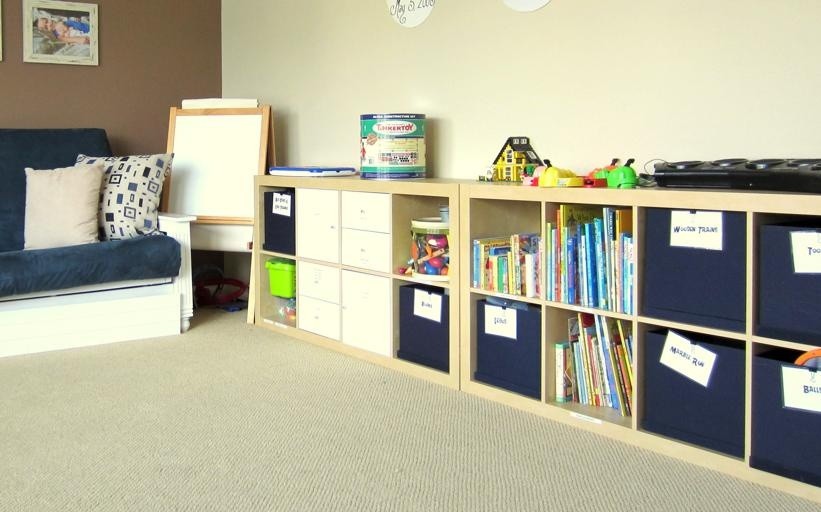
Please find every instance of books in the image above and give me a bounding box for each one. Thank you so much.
[472,201,634,424]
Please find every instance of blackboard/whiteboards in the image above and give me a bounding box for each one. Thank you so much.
[159,105,270,225]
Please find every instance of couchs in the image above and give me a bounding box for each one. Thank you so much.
[0,128,198,360]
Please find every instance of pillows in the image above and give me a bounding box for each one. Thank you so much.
[23,161,105,250]
[73,152,174,241]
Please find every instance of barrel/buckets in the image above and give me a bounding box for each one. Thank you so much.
[360,113,428,181]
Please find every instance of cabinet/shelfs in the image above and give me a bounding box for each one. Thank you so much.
[254,175,460,393]
[460,179,821,504]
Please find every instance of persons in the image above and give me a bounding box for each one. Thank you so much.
[34,16,90,45]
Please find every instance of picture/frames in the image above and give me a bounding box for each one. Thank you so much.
[21,1,99,67]
